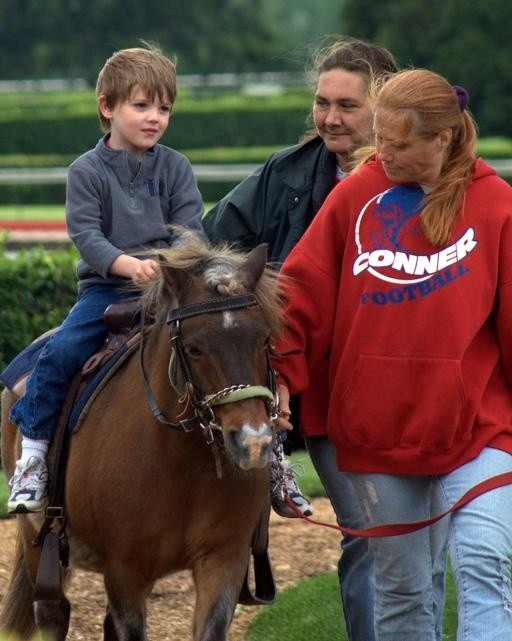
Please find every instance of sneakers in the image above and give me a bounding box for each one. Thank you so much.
[271,462,313,518]
[9,456,48,513]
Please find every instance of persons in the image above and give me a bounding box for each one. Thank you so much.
[200,39,447,640]
[264,69,511,641]
[6,39,314,518]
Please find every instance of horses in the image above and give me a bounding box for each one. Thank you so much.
[0,222,298,641]
